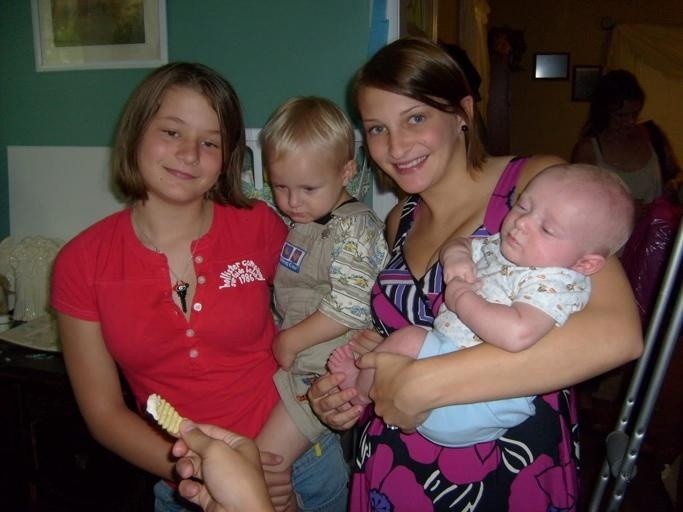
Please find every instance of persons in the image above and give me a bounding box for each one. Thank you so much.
[572,69,683,454]
[48,60,352,510]
[172,420,273,511]
[327,164,637,447]
[307,38,646,512]
[254,95,393,509]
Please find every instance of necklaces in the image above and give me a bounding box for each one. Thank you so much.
[129,205,214,313]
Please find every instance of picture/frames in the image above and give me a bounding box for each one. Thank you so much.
[28,1,170,73]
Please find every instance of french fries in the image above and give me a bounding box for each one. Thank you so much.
[147,393,185,436]
